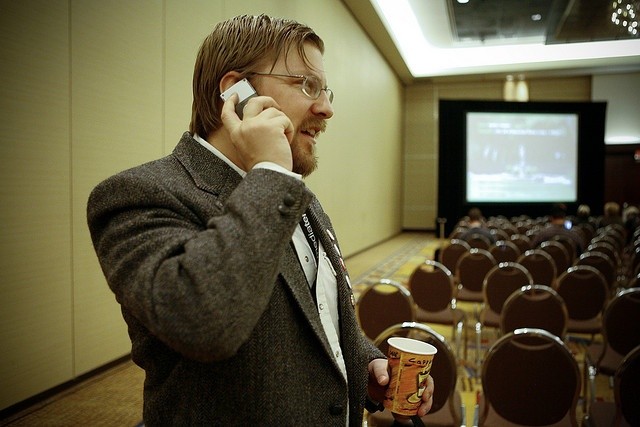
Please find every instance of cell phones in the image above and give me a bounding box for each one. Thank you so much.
[220,76,266,125]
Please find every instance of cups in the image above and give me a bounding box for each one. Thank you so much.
[386,336,438,415]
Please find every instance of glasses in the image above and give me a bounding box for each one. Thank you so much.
[250,72,334,104]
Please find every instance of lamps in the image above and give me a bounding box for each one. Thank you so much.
[502,75,531,104]
[610,0,640,38]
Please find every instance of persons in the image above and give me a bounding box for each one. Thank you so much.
[83,12,437,425]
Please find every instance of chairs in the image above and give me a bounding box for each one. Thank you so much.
[500,285,570,344]
[552,266,610,366]
[440,210,626,249]
[480,330,593,426]
[476,264,534,361]
[455,249,496,302]
[583,346,639,427]
[364,323,467,427]
[449,249,497,341]
[512,250,557,300]
[405,261,467,342]
[583,287,639,412]
[441,238,470,276]
[573,252,614,291]
[356,280,417,342]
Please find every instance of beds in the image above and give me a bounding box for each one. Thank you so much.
[623,226,639,285]
